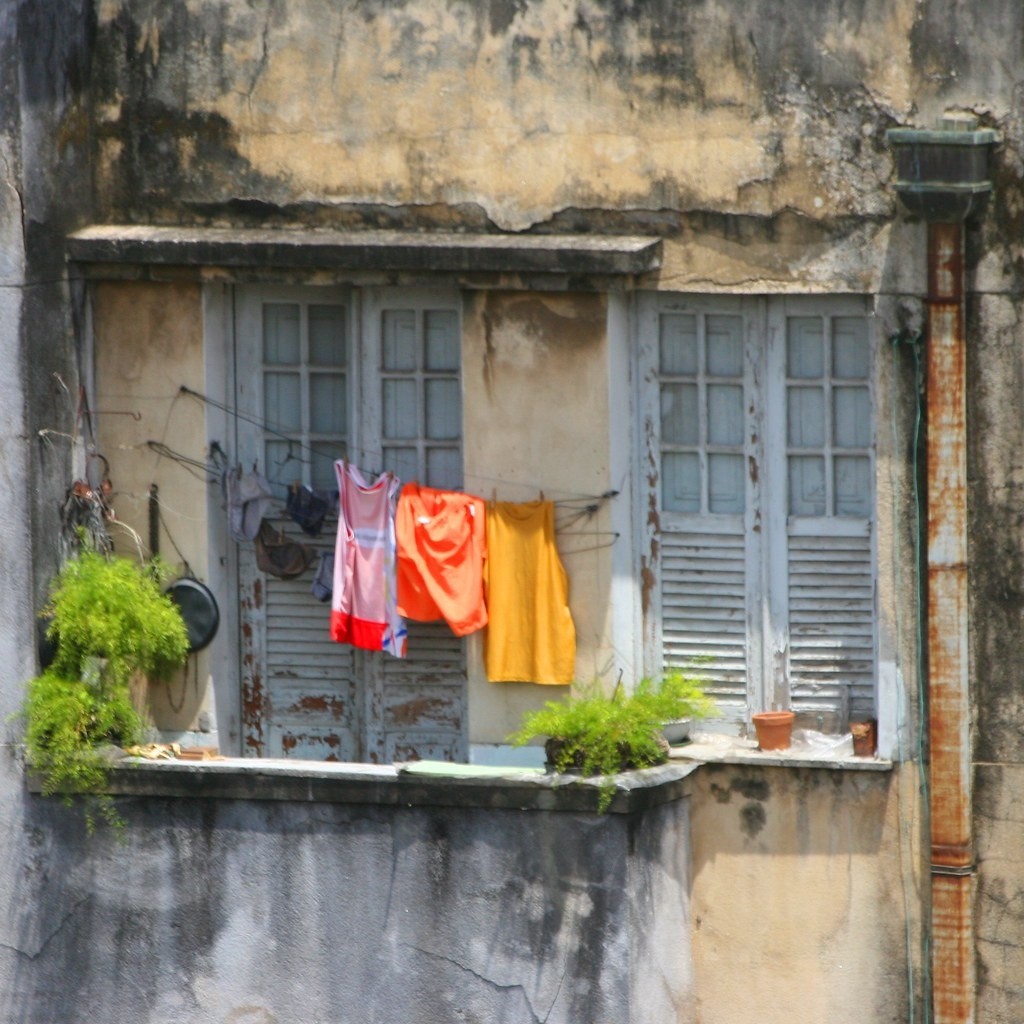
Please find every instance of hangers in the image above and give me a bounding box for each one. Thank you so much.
[267,435,620,554]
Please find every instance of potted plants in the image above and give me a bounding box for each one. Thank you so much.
[510,659,670,817]
[631,652,729,748]
[4,527,191,845]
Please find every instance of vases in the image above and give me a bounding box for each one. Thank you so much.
[751,710,793,751]
